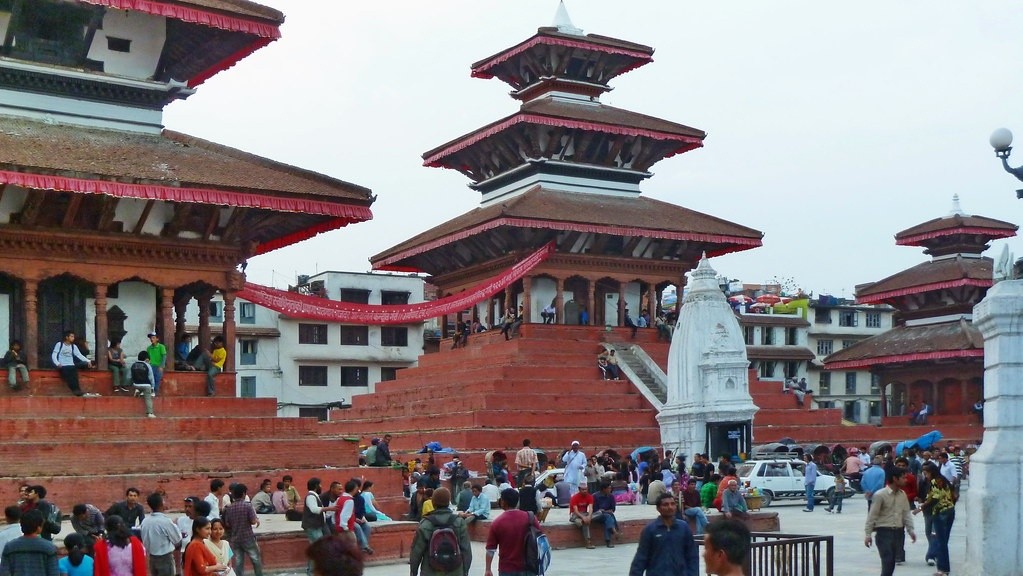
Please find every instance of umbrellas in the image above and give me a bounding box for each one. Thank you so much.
[727,295,791,315]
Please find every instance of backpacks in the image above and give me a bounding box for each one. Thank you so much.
[422,511,462,572]
[46,502,61,534]
[522,511,551,576]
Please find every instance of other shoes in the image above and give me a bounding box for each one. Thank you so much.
[803,507,812,512]
[120,386,129,392]
[144,413,157,418]
[584,538,596,549]
[927,557,937,566]
[607,541,614,548]
[113,386,120,392]
[134,389,140,397]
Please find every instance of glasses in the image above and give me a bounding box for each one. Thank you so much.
[184,498,193,502]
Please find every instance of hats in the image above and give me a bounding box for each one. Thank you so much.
[432,487,451,507]
[555,474,565,480]
[371,438,382,445]
[147,331,157,338]
[572,440,580,445]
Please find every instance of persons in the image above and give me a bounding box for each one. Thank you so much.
[789,377,812,403]
[108,330,226,417]
[629,493,700,576]
[450,306,523,350]
[52,331,100,397]
[484,488,541,576]
[845,440,983,576]
[971,400,984,424]
[597,349,621,381]
[824,469,845,513]
[401,439,750,550]
[542,296,676,344]
[802,453,816,512]
[3,340,31,389]
[408,487,474,576]
[359,434,398,467]
[0,475,394,576]
[907,401,933,425]
[702,518,752,576]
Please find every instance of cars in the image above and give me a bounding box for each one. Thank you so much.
[735,459,853,508]
[513,467,565,494]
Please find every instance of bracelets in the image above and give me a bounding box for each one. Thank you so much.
[918,506,922,510]
